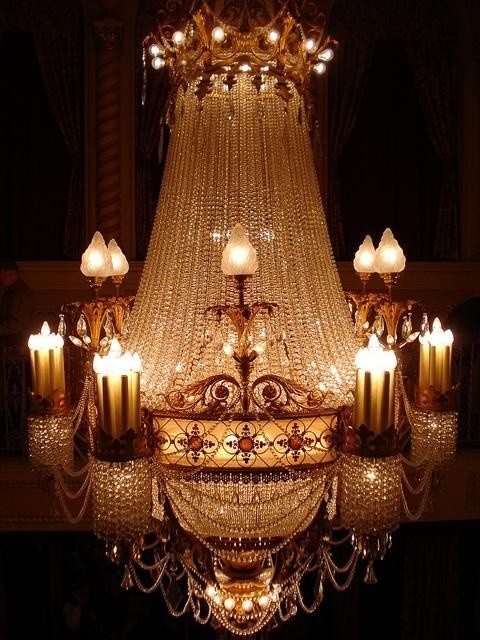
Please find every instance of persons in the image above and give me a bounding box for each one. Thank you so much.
[445,297,480,447]
[0,259,36,431]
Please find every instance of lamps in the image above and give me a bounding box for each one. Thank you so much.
[28,1,463,637]
